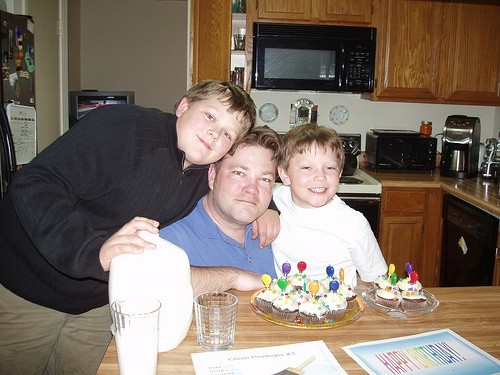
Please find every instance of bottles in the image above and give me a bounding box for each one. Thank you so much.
[420,121,433,135]
[237,28,245,50]
[231,67,244,89]
[108,229,194,353]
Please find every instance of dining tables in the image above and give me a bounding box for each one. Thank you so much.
[95,286,500,375]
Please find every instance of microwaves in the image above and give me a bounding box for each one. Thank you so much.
[365,129,437,174]
[252,22,377,92]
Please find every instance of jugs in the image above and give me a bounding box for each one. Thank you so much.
[443,148,466,171]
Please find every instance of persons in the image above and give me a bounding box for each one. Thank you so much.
[270,122,389,289]
[158,125,278,305]
[0,78,282,375]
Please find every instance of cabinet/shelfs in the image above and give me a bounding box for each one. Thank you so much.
[362,0,500,107]
[379,186,440,286]
[244,0,381,93]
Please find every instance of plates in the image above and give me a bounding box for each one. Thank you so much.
[362,286,438,317]
[250,288,365,330]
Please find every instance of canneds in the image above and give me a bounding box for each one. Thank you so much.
[420,120,432,135]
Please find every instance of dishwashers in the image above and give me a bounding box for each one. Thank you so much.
[440,195,497,287]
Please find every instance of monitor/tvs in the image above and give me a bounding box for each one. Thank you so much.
[69,91,135,127]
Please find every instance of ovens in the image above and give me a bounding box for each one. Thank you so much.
[339,197,381,241]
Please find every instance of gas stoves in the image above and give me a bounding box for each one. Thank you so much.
[274,132,382,195]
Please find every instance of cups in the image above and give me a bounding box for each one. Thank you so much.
[195,291,238,351]
[111,298,162,375]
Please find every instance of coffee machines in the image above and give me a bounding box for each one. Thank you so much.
[439,115,482,179]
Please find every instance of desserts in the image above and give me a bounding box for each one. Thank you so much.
[374,262,428,312]
[254,262,358,325]
